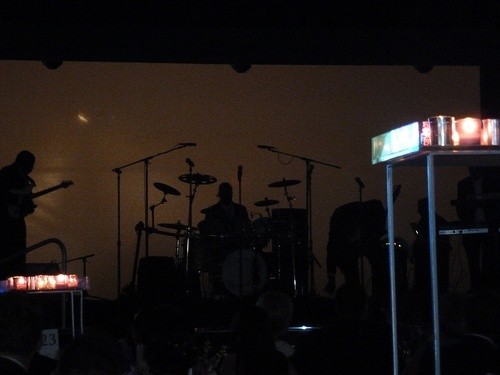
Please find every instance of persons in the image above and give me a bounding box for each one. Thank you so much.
[202,184,250,250]
[0,150,35,279]
[411,198,452,332]
[0,310,44,375]
[54,290,297,375]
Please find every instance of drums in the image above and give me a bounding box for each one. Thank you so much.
[184,217,272,277]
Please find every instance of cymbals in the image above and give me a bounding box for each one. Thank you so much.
[159,223,198,231]
[253,200,280,207]
[153,182,181,196]
[268,180,301,188]
[179,174,218,185]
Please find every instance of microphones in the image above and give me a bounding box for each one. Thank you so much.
[186,158,194,167]
[238,165,242,178]
[257,145,277,149]
[355,177,365,188]
[178,142,196,146]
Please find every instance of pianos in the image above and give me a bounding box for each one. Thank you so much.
[439,227,490,235]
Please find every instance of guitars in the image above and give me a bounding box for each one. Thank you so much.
[384,184,402,215]
[8,180,74,219]
